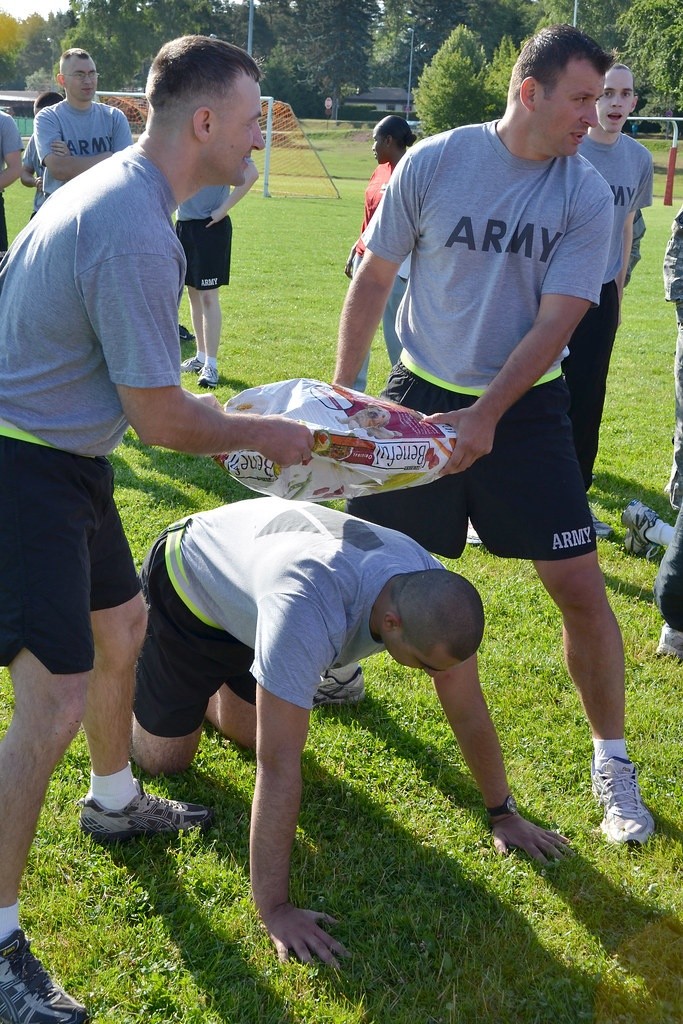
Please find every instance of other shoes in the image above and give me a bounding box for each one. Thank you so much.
[589,510,615,542]
[467,518,482,545]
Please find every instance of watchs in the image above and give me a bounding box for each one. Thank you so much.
[487,796,517,817]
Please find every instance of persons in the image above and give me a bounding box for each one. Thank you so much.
[21,92,63,221]
[563,64,653,536]
[353,116,417,393]
[179,158,259,388]
[130,497,575,971]
[314,24,652,845]
[0,111,25,250]
[622,208,683,665]
[33,48,196,342]
[1,36,314,1024]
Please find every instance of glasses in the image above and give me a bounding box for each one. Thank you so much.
[62,72,99,80]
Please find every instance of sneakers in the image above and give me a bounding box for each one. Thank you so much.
[0,929,88,1024]
[657,623,683,665]
[591,756,654,850]
[312,667,365,707]
[181,356,205,373]
[622,500,662,561]
[81,778,215,845]
[178,324,196,341]
[197,364,219,388]
